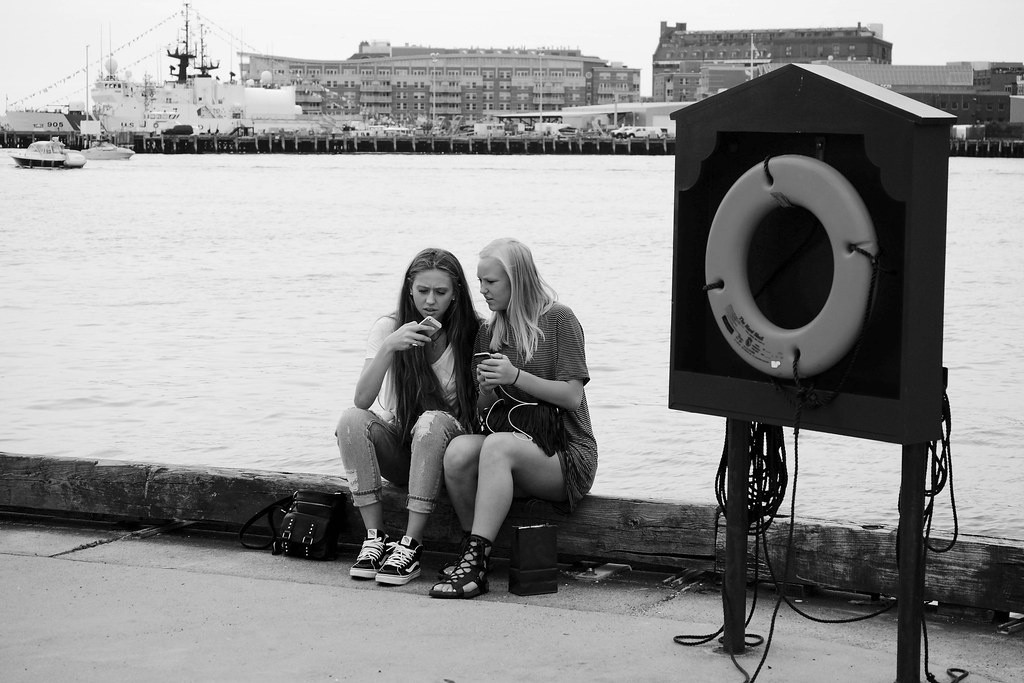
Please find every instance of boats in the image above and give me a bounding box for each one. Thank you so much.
[80,138,135,160]
[6,135,86,168]
[90,2,364,137]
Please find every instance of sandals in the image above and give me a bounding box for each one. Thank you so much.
[437,537,468,579]
[428,534,492,599]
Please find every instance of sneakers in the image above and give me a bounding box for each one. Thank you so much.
[350,529,391,578]
[375,535,424,584]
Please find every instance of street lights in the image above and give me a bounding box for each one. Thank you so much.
[85,43,94,120]
[430,52,440,132]
[538,51,546,136]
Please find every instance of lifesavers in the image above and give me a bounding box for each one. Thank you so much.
[702,156,881,382]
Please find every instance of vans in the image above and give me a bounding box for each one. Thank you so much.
[610,124,649,139]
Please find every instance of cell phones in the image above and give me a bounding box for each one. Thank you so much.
[474,352,492,365]
[412,316,442,346]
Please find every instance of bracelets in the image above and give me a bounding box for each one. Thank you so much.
[478,384,494,397]
[511,368,520,387]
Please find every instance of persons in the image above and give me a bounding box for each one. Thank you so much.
[429,236,597,599]
[333,248,486,588]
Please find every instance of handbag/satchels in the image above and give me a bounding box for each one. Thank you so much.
[480,383,573,456]
[509,519,559,597]
[277,489,348,560]
